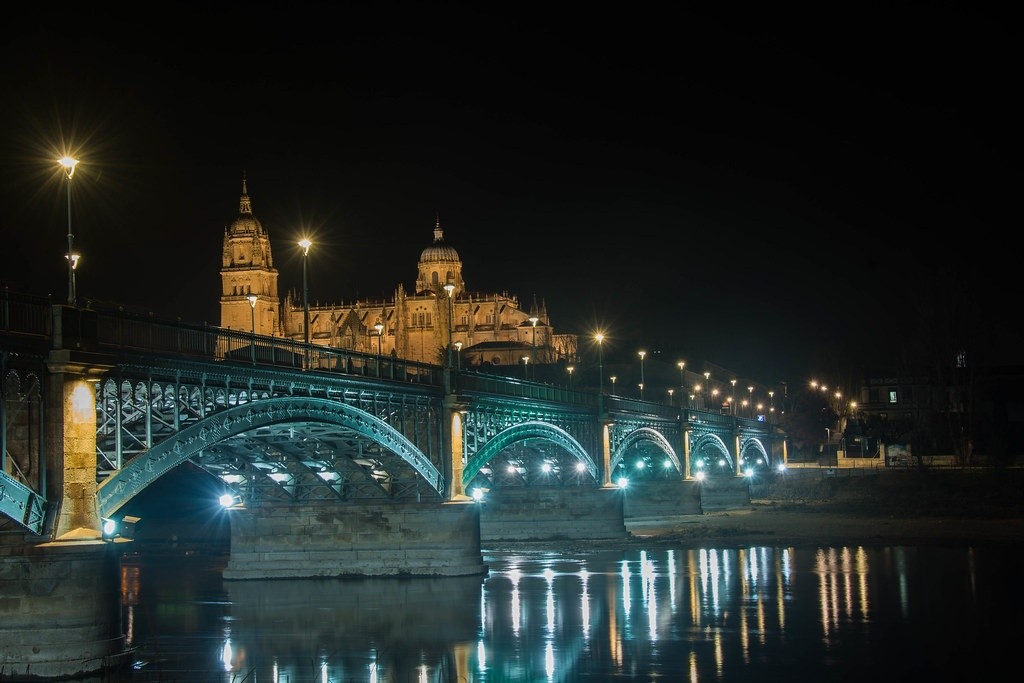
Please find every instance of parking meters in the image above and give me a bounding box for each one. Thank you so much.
[769,391,774,403]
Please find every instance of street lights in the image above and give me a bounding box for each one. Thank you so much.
[595,333,604,394]
[824,427,831,467]
[747,387,753,420]
[375,322,384,355]
[444,283,456,368]
[63,254,79,306]
[454,341,462,370]
[610,376,616,395]
[638,384,643,400]
[522,356,529,381]
[567,367,574,390]
[678,362,684,406]
[297,237,314,369]
[704,373,710,414]
[667,389,674,407]
[727,397,732,416]
[638,352,646,400]
[57,153,80,310]
[529,317,538,382]
[731,380,737,415]
[247,292,258,364]
[690,395,694,410]
